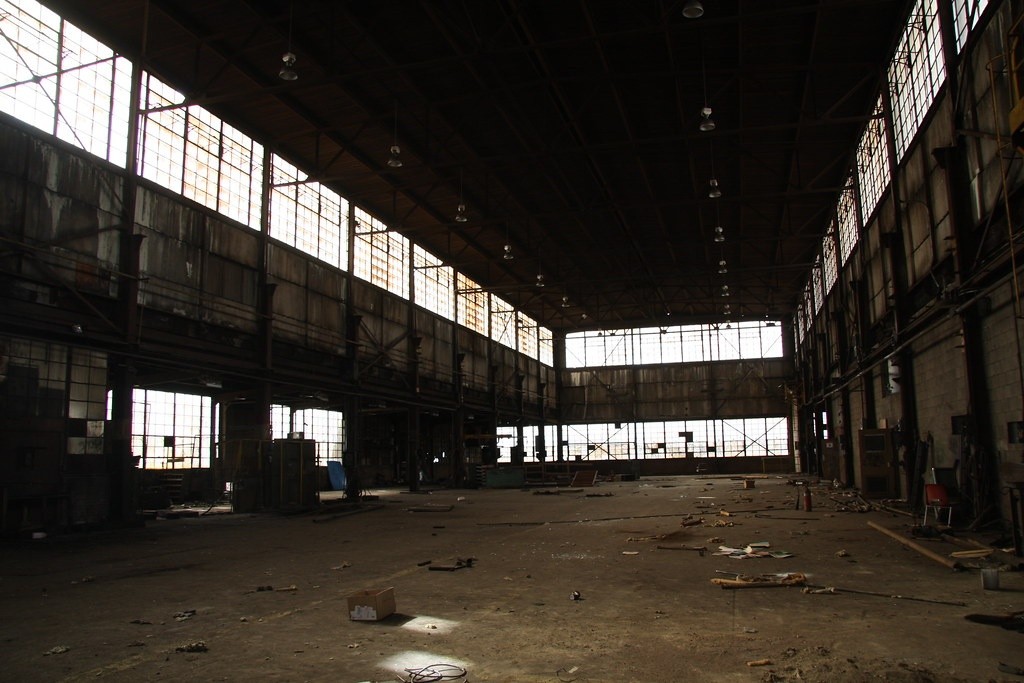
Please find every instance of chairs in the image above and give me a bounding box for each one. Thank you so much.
[923,483,964,528]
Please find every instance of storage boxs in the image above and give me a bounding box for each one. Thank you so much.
[347,587,396,621]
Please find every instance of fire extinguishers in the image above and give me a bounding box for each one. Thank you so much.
[795,479,812,512]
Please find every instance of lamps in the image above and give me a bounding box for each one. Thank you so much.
[535,273,545,288]
[699,107,716,131]
[503,243,514,262]
[682,0,704,18]
[721,285,730,296]
[713,225,726,242]
[723,304,731,314]
[725,319,732,330]
[708,177,721,198]
[561,295,571,308]
[278,52,298,81]
[454,201,468,223]
[386,146,403,168]
[717,260,727,274]
